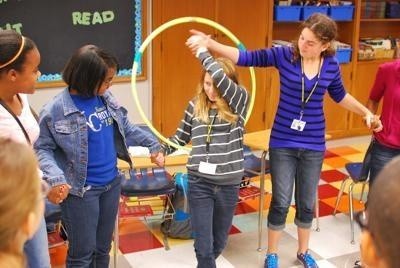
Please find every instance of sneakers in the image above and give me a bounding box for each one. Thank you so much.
[297,250,319,268]
[264,253,278,268]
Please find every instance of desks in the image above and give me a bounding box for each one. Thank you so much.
[240,129,332,252]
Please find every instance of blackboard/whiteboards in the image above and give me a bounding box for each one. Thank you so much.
[0,0,147,89]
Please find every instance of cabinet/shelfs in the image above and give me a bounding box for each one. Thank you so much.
[152,1,268,143]
[268,0,357,143]
[350,0,400,141]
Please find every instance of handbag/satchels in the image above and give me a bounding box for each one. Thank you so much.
[160,172,192,240]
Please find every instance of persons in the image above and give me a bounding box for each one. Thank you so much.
[358,154,399,268]
[33,43,165,268]
[159,34,251,268]
[0,29,71,267]
[185,12,385,268]
[0,137,46,268]
[356,60,400,228]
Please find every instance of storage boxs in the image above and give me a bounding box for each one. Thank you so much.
[329,4,355,21]
[273,3,303,23]
[332,48,351,65]
[301,3,329,21]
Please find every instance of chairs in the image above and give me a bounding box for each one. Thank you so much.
[112,121,177,252]
[332,143,374,245]
